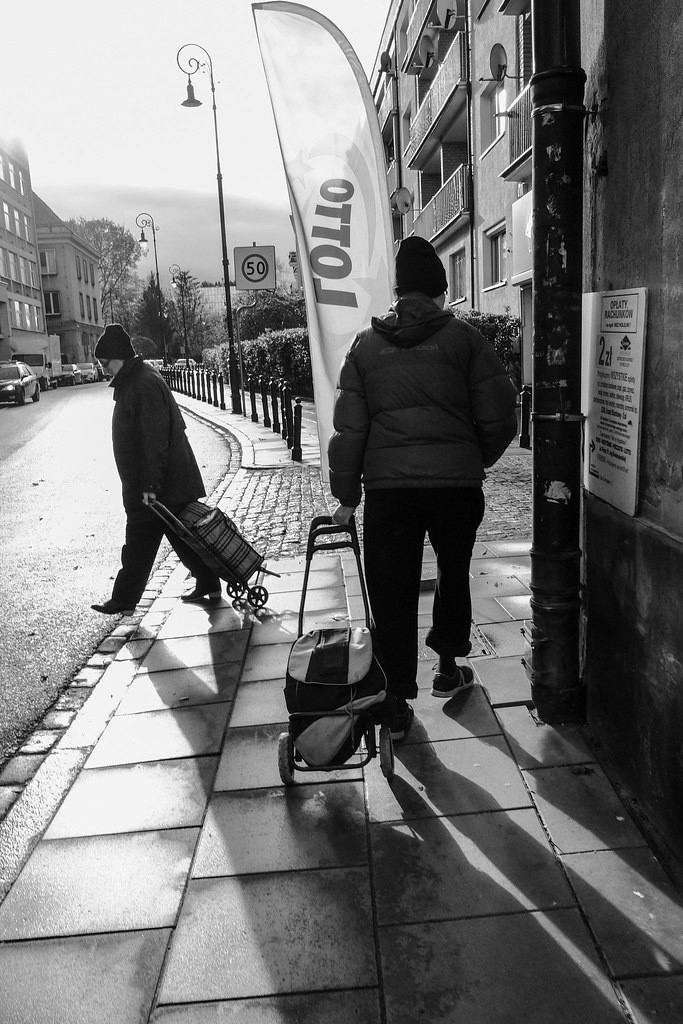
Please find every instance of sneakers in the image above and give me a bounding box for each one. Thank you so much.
[429,662,475,698]
[388,702,414,739]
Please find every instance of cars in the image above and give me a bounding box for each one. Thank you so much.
[96,363,114,382]
[58,364,83,387]
[77,363,99,383]
[141,360,165,371]
[175,358,196,369]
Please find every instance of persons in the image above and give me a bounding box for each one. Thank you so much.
[90,323,222,616]
[329,238,520,740]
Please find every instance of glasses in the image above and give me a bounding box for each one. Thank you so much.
[443,289,448,298]
[97,359,110,368]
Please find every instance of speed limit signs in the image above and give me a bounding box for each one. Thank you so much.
[232,245,277,291]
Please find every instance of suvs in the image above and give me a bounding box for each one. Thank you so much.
[0,360,41,406]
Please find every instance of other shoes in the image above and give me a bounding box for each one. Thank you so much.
[90,599,136,616]
[181,580,222,602]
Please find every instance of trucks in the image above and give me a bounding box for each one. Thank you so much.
[9,335,62,391]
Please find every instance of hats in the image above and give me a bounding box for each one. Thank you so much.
[94,323,136,360]
[392,235,448,298]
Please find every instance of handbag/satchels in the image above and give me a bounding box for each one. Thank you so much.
[284,626,389,768]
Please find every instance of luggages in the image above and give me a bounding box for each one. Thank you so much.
[148,498,268,607]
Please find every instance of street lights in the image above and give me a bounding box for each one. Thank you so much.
[176,42,244,415]
[136,212,168,368]
[169,264,191,371]
